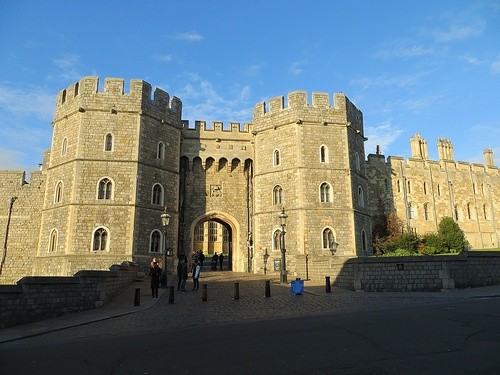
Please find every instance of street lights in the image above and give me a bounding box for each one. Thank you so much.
[160,207,172,288]
[278,207,289,283]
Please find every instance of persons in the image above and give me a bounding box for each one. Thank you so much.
[149,258,162,299]
[177,258,188,292]
[212,252,223,270]
[195,250,205,268]
[192,260,200,290]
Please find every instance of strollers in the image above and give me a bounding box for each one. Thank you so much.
[210,259,217,270]
